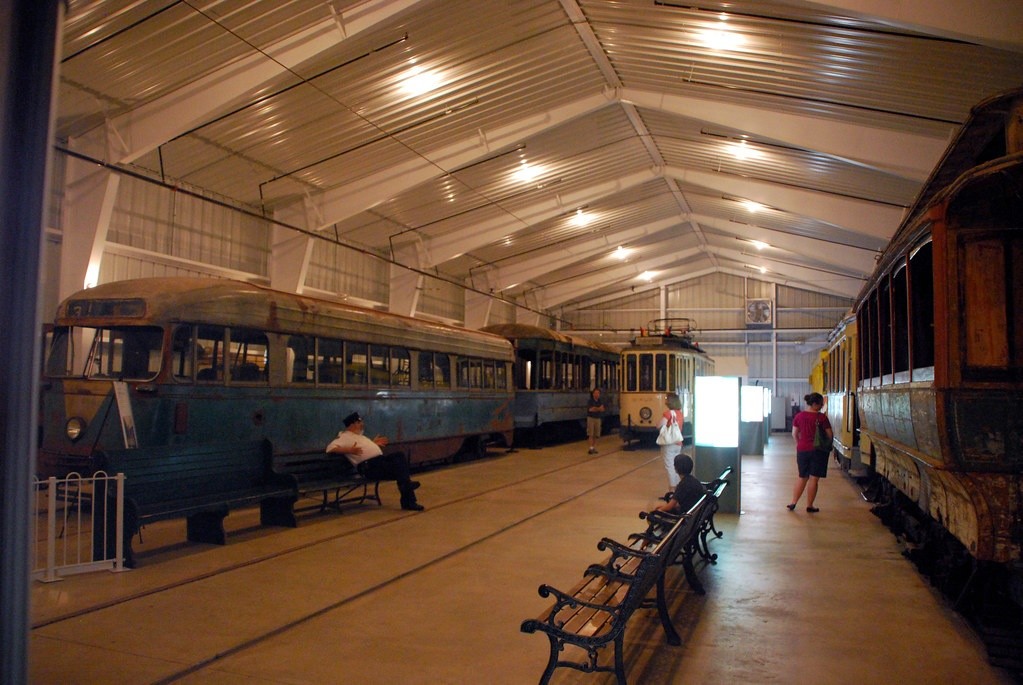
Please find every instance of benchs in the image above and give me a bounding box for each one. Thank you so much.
[90,437,381,570]
[518,467,736,685]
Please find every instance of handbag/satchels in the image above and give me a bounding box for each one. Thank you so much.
[655,410,684,446]
[813,412,833,452]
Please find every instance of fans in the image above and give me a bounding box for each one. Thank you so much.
[747,298,773,324]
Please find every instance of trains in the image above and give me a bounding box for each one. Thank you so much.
[810,86,1022,610]
[616,314,715,449]
[37,268,623,529]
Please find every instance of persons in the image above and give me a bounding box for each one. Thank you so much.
[326,412,424,511]
[586,389,605,454]
[787,391,833,513]
[656,392,683,493]
[655,454,704,514]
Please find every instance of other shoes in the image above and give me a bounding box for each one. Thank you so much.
[807,507,819,512]
[659,493,673,499]
[594,449,599,453]
[588,449,594,454]
[401,502,424,510]
[410,481,420,489]
[786,505,795,510]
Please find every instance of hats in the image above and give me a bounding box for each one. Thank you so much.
[343,412,363,426]
